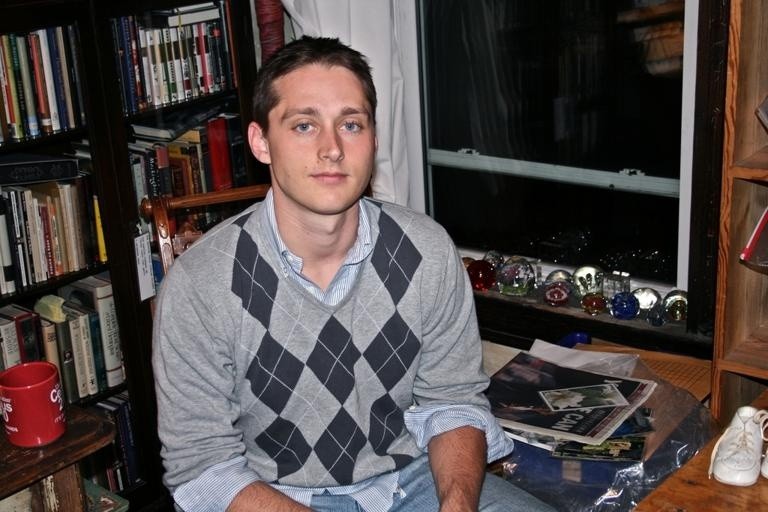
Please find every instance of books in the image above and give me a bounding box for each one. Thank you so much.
[2,277,125,407]
[1,140,103,296]
[116,1,238,116]
[1,23,86,143]
[79,397,132,511]
[130,113,245,254]
[480,347,658,459]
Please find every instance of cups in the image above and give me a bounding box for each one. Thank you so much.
[1,362,65,447]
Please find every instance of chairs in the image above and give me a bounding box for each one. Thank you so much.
[141,185,288,320]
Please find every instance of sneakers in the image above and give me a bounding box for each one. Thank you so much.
[707,406,762,487]
[760,414,768,479]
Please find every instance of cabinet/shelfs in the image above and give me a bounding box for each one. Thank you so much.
[709,0,767,454]
[1,0,268,512]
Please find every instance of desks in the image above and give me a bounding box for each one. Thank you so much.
[627,423,765,511]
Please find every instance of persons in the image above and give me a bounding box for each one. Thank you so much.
[152,34,558,510]
